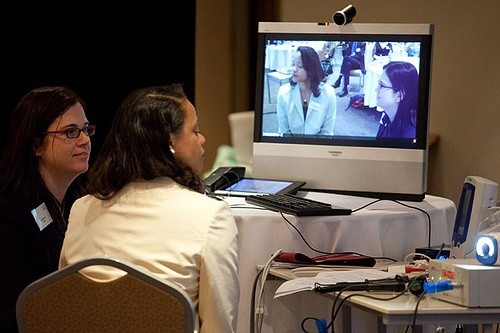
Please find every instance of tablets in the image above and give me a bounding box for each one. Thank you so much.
[214,176,306,197]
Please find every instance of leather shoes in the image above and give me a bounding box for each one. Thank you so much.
[331,80,340,88]
[338,89,348,97]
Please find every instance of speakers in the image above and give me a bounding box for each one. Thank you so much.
[451,176,500,259]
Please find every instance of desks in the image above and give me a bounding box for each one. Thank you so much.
[256,260,500,333]
[202,195,457,333]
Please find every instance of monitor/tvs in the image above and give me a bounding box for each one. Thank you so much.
[252,21,434,202]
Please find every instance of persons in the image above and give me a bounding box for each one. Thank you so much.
[277,41,418,138]
[62,84,240,333]
[0,87,91,333]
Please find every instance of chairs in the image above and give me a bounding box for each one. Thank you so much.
[16,256,195,333]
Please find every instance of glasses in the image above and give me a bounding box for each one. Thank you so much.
[45,125,95,138]
[378,82,395,89]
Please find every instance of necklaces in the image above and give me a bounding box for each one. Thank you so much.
[303,100,307,104]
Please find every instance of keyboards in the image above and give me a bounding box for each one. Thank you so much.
[246,193,352,216]
